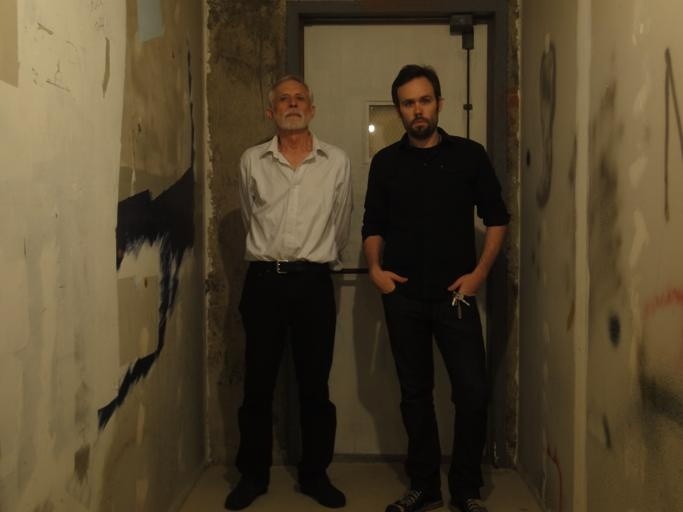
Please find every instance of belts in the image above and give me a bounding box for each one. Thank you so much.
[274,258,288,274]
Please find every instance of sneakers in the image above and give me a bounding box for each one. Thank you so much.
[448,492,488,512]
[299,476,346,508]
[225,474,269,510]
[386,488,442,512]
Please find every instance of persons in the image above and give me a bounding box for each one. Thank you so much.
[362,63,510,507]
[219,77,355,512]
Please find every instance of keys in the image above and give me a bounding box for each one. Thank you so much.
[451,285,471,320]
